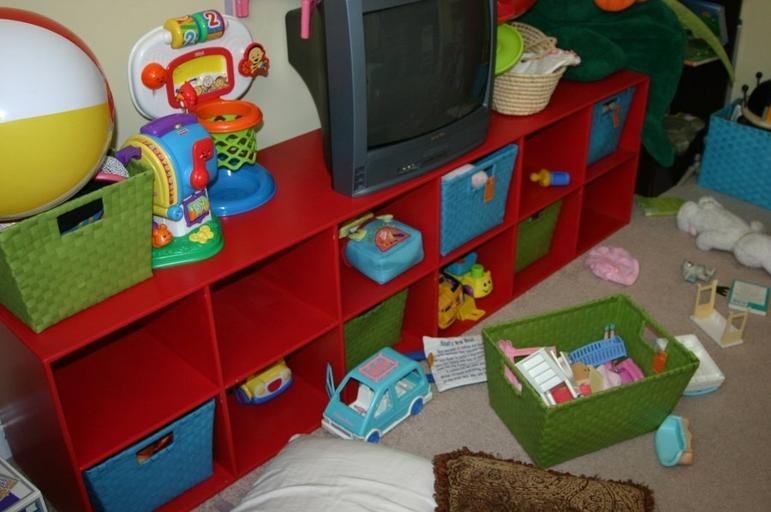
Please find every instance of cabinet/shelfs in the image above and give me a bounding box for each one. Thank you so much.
[0,68,653,511]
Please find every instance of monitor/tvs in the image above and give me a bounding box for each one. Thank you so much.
[286,0,496,199]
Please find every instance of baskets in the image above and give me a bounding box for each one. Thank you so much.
[439,143,519,260]
[80,396,218,512]
[586,87,640,170]
[491,20,570,117]
[479,292,702,472]
[695,95,771,210]
[512,200,564,277]
[0,149,155,335]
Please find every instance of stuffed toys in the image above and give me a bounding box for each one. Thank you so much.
[676,195,771,276]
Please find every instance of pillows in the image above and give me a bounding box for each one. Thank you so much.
[427,446,656,510]
[229,425,439,510]
